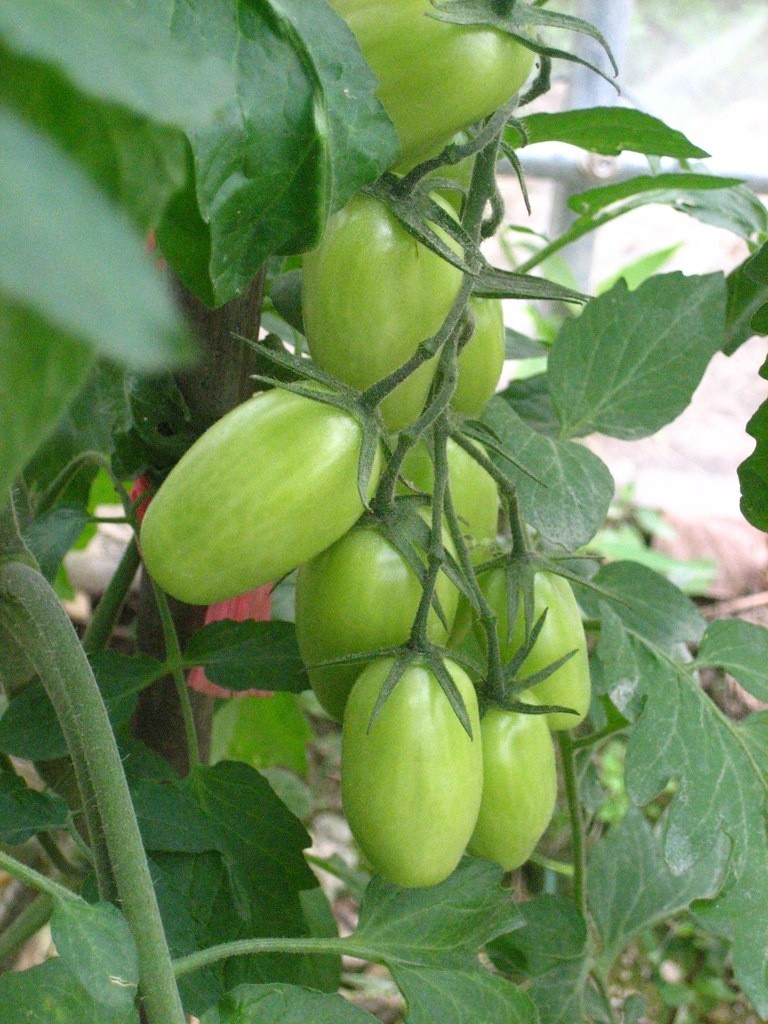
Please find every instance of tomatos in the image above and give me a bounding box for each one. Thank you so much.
[138,0,591,888]
